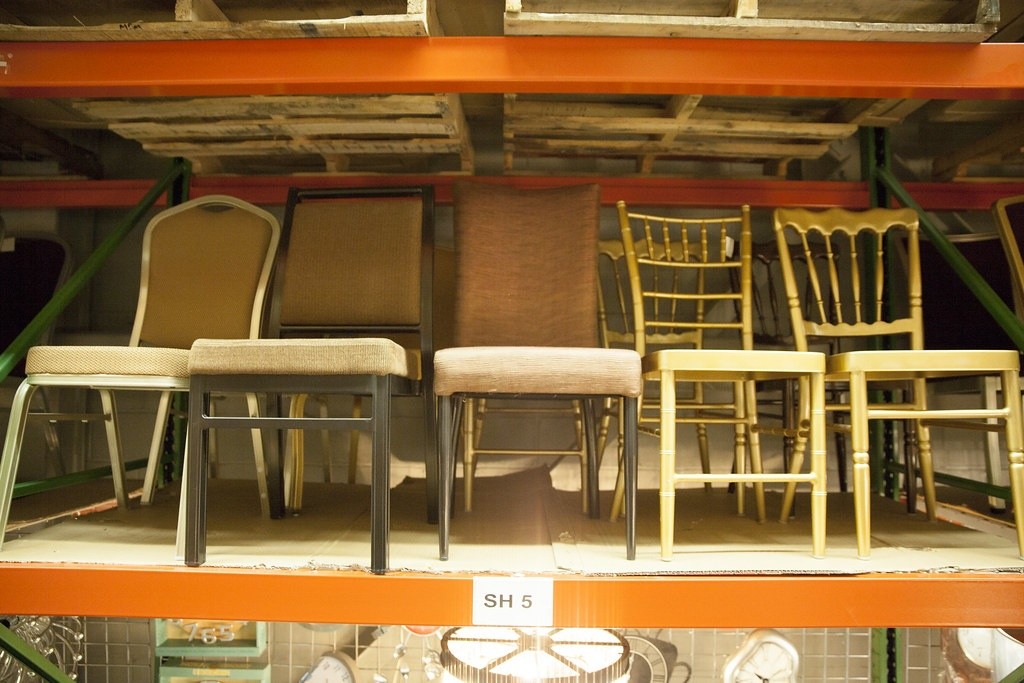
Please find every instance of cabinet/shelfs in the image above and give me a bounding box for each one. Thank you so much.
[0,37,1024,631]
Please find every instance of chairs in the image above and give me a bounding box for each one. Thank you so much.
[0,231,77,477]
[615,199,828,560]
[726,242,918,515]
[891,232,1024,516]
[180,184,439,576]
[772,208,1024,562]
[594,237,714,497]
[0,194,273,562]
[431,177,643,560]
